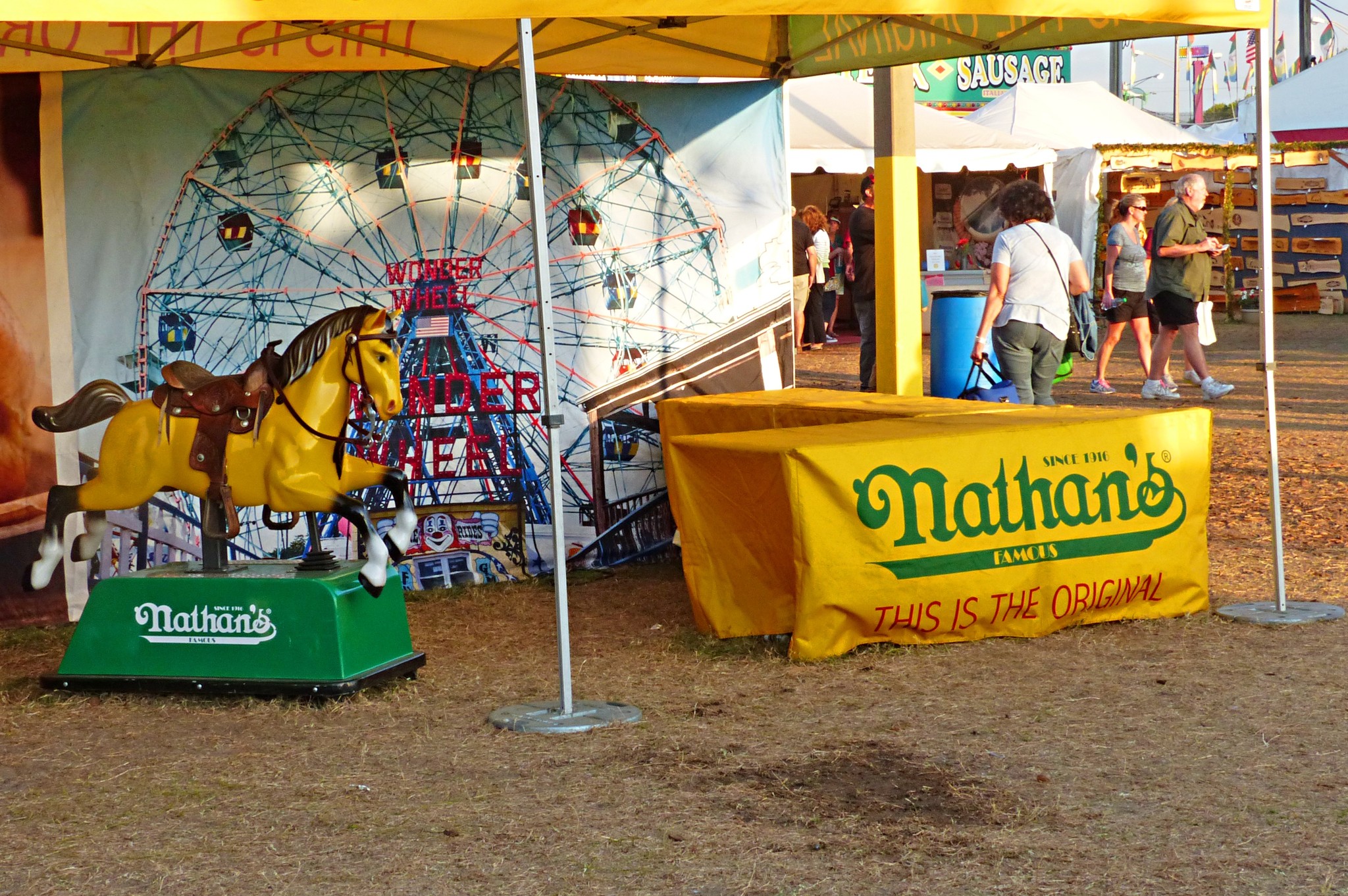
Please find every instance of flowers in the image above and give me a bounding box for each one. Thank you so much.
[954,238,972,268]
[1235,286,1259,309]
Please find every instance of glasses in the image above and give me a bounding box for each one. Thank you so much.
[1132,206,1148,212]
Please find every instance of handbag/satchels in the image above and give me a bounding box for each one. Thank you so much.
[821,276,842,292]
[1065,312,1081,353]
[957,353,1020,404]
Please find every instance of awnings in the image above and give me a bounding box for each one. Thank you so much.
[1191,44,1348,150]
[784,80,1060,227]
[967,78,1241,325]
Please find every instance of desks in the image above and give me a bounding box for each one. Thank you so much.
[653,386,1213,665]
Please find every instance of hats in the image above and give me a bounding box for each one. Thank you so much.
[791,174,875,235]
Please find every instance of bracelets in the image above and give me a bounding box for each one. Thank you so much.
[975,334,988,346]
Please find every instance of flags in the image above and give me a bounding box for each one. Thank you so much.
[1183,11,1340,98]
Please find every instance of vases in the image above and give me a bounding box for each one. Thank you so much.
[960,255,967,270]
[1240,309,1259,324]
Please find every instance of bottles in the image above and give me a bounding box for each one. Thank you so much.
[1100,297,1127,310]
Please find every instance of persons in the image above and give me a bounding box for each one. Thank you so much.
[789,203,818,355]
[819,211,844,337]
[847,173,878,394]
[1151,197,1207,395]
[821,210,839,346]
[842,227,862,341]
[1140,171,1239,404]
[971,178,1095,403]
[798,203,832,350]
[1087,191,1152,395]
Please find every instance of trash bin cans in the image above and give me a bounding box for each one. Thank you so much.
[931,290,1001,400]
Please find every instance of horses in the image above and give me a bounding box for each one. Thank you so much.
[20,306,419,599]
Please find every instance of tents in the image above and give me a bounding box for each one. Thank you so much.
[0,3,1346,738]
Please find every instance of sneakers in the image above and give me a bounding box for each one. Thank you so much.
[793,325,878,392]
[1201,380,1234,402]
[1183,370,1202,386]
[1162,374,1178,392]
[1089,380,1115,394]
[1141,379,1180,400]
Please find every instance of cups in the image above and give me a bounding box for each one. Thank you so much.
[837,252,843,268]
